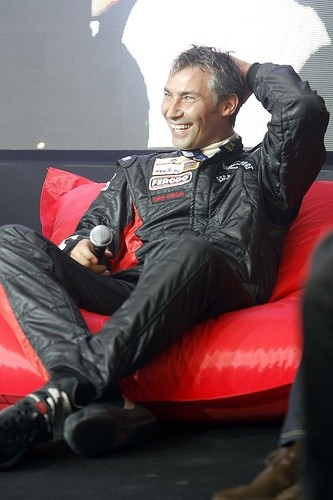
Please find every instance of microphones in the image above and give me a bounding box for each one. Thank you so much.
[90,225,113,264]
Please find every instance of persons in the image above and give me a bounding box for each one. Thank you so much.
[209,235,306,500]
[0,43,330,473]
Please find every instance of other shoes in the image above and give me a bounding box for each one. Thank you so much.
[64,390,157,453]
[0,376,82,472]
[211,440,303,500]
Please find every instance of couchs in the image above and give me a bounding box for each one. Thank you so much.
[0,179,333,422]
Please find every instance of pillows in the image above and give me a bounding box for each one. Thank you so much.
[39,166,145,275]
